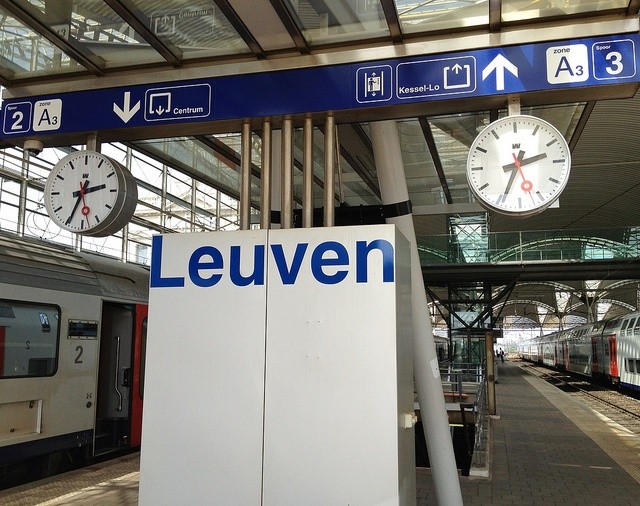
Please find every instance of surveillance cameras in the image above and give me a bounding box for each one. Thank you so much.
[24,140,43,157]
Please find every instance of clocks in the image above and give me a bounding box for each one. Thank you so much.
[45,149,121,234]
[467,113,573,219]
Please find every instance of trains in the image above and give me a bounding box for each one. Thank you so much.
[0,228,448,477]
[516,313,640,393]
